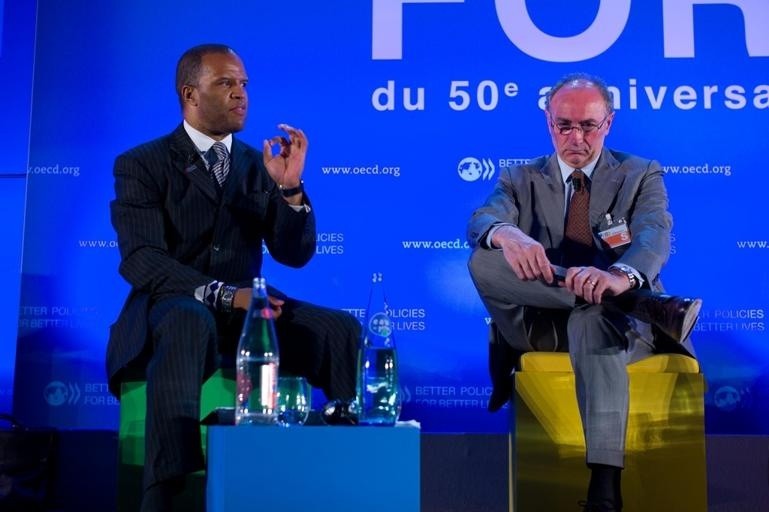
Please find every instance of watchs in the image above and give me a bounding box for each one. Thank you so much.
[609,265,636,289]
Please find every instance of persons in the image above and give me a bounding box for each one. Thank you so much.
[465,71,703,509]
[105,42,361,511]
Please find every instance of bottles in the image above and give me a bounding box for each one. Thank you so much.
[355,270,402,427]
[233,275,280,428]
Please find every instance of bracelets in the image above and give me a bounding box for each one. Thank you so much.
[221,283,237,312]
[279,178,304,197]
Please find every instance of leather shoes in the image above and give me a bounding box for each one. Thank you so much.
[577,496,623,512]
[645,291,702,343]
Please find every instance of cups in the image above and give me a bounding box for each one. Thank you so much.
[273,374,311,427]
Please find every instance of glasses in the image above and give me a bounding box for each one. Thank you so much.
[545,111,615,135]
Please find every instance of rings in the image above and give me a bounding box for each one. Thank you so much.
[587,280,597,286]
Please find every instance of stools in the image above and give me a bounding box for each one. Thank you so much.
[118,369,314,479]
[511,348,703,459]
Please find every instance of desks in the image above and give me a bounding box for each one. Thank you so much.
[208,420,421,510]
[199,407,423,512]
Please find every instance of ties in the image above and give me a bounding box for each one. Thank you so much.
[210,142,231,185]
[565,170,593,246]
